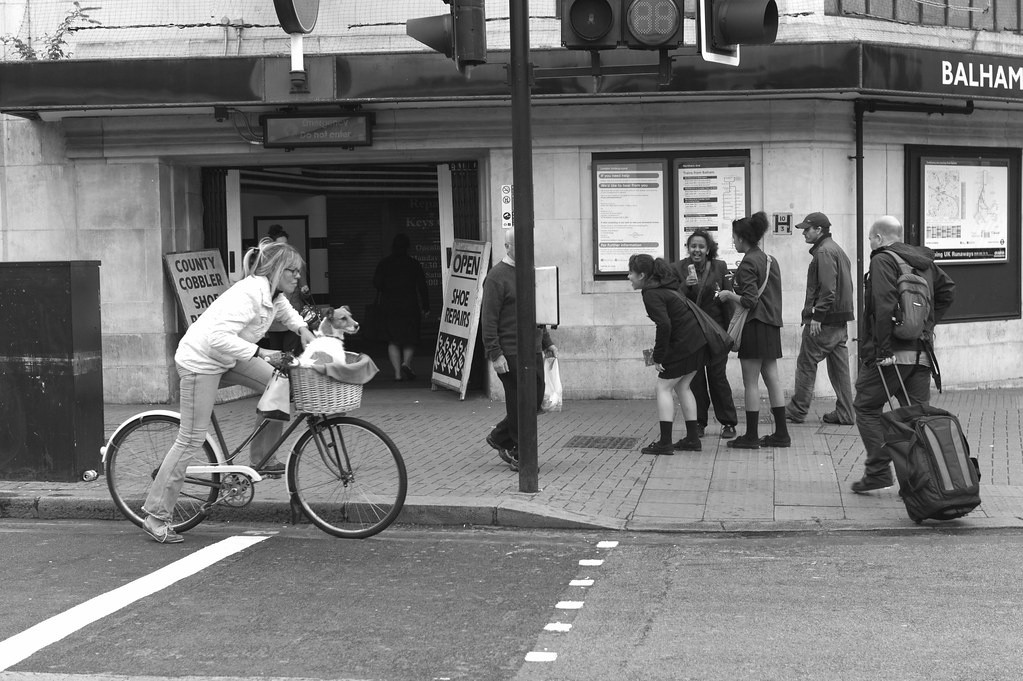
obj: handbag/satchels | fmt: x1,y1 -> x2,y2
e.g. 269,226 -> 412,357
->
256,353 -> 291,422
539,357 -> 562,412
684,297 -> 734,357
726,304 -> 751,352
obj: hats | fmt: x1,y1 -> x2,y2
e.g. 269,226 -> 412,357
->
795,212 -> 831,229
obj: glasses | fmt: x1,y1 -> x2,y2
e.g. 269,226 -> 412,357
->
283,268 -> 302,277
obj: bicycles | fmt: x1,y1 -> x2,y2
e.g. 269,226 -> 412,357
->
100,349 -> 408,540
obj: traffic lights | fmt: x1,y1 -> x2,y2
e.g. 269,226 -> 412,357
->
406,0 -> 488,76
696,0 -> 779,67
560,0 -> 622,50
622,0 -> 685,51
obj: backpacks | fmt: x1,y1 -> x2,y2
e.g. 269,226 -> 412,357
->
879,246 -> 931,343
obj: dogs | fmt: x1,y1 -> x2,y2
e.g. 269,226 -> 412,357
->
296,305 -> 360,365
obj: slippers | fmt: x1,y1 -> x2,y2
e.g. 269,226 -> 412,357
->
401,363 -> 416,379
395,377 -> 403,382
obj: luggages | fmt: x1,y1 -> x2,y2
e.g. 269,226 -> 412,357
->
877,358 -> 982,524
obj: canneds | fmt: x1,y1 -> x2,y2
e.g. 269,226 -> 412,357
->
83,469 -> 98,482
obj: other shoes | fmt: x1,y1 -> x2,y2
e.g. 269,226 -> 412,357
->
850,475 -> 893,492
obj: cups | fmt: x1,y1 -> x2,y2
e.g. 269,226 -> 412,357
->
643,349 -> 654,366
725,274 -> 733,290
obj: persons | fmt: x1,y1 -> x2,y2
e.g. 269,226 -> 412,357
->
627,254 -> 705,454
482,227 -> 558,473
373,233 -> 430,383
267,225 -> 310,402
769,212 -> 856,425
672,230 -> 738,438
141,236 -> 316,544
851,216 -> 956,493
719,211 -> 792,448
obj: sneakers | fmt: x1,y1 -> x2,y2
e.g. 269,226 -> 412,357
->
486,434 -> 512,464
641,441 -> 674,455
721,425 -> 736,438
727,436 -> 761,449
760,435 -> 791,447
141,516 -> 184,543
249,461 -> 286,474
674,437 -> 701,451
697,423 -> 704,437
823,411 -> 840,424
498,447 -> 540,473
771,407 -> 802,424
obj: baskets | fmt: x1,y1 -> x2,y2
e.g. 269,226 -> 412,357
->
287,351 -> 363,415
